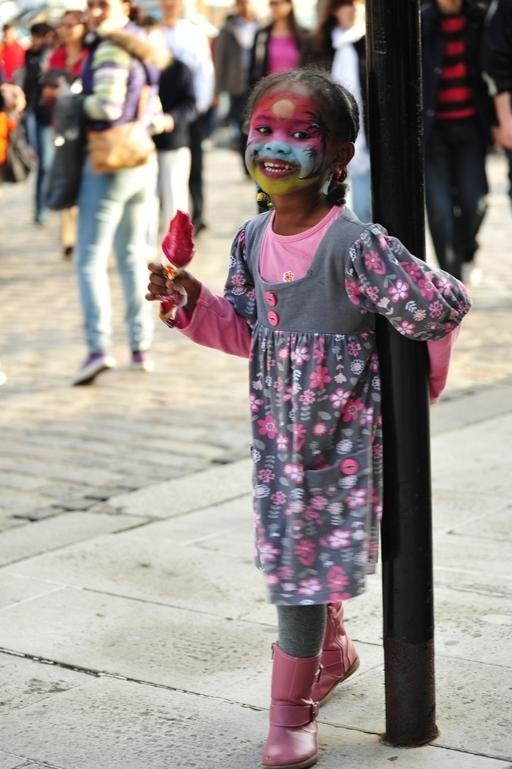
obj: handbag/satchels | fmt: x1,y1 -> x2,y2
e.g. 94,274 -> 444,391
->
83,85 -> 155,173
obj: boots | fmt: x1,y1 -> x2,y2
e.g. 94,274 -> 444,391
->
311,601 -> 360,704
261,642 -> 319,768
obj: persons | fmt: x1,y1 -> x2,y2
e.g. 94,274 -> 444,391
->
2,2 -> 512,384
145,69 -> 472,766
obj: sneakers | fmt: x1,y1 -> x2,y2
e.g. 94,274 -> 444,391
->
71,353 -> 113,386
130,351 -> 150,369
